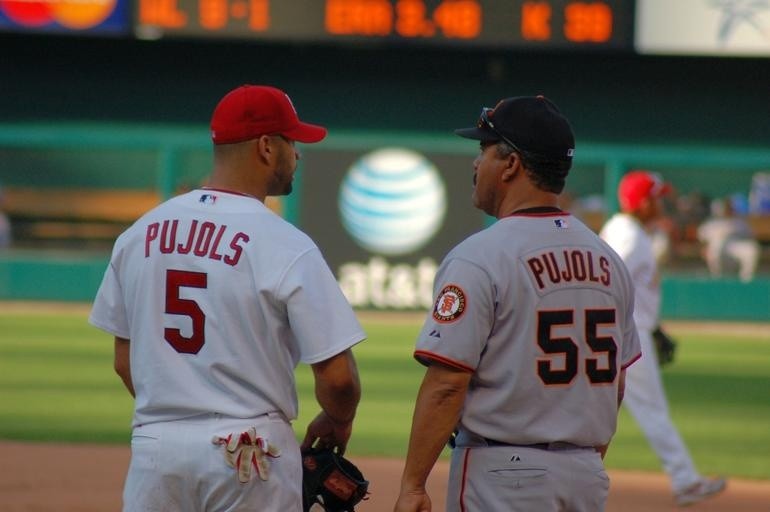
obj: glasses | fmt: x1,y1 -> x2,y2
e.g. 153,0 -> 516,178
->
477,107 -> 525,156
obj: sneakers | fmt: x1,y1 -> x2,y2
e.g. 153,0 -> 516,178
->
673,475 -> 725,506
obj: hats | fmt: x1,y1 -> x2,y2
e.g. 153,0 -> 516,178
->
210,85 -> 328,145
618,170 -> 672,211
453,93 -> 574,170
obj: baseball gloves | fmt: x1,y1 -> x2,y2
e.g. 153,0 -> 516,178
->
653,327 -> 675,362
301,446 -> 371,511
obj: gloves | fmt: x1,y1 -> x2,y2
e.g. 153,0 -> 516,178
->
300,447 -> 370,512
652,328 -> 675,366
211,426 -> 282,484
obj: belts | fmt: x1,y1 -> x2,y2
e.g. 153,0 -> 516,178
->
478,433 -> 553,450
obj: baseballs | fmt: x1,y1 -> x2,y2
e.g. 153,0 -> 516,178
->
336,147 -> 447,256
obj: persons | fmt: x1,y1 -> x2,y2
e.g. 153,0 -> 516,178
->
596,168 -> 732,507
657,170 -> 769,287
81,82 -> 371,510
388,94 -> 646,512
